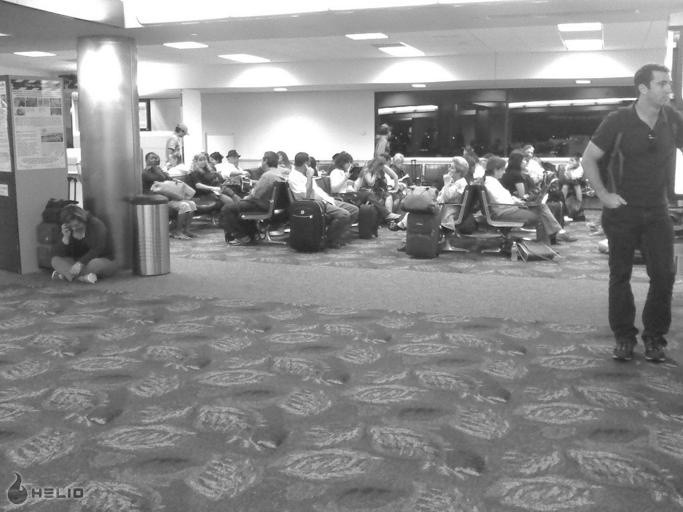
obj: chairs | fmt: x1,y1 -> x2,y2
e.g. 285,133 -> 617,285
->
65,157 -> 588,260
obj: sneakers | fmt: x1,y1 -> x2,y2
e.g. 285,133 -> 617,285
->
79,273 -> 98,284
227,234 -> 253,247
49,270 -> 64,282
610,336 -> 635,362
388,223 -> 407,231
555,230 -> 579,243
172,230 -> 201,240
642,339 -> 667,363
395,244 -> 407,252
563,216 -> 574,222
384,213 -> 401,220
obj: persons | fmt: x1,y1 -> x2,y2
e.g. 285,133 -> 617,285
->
49,205 -> 113,284
583,64 -> 683,363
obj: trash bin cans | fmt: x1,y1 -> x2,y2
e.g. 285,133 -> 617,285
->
132,194 -> 170,277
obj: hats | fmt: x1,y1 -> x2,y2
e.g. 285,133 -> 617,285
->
175,123 -> 192,138
225,149 -> 241,159
